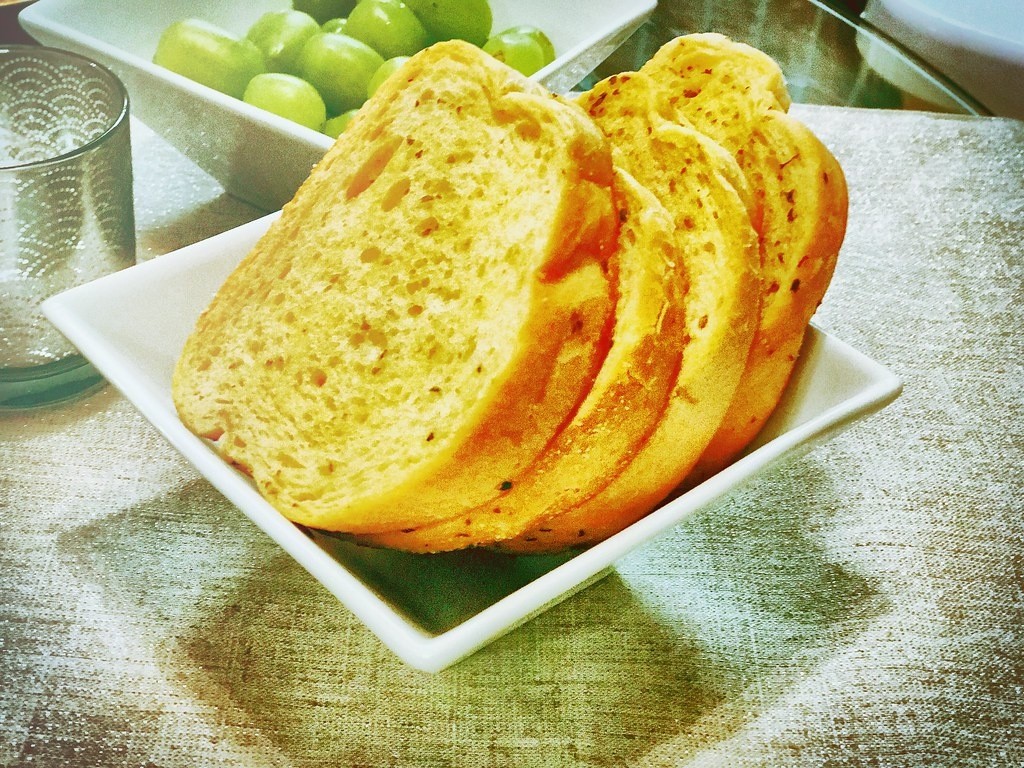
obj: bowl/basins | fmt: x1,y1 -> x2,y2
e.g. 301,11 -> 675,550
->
20,0 -> 661,205
38,208 -> 902,674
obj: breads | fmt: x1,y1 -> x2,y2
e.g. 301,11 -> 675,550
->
336,31 -> 848,554
172,40 -> 621,534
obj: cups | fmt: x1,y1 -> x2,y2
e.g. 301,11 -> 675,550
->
0,43 -> 135,413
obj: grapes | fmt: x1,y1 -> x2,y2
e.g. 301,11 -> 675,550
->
151,0 -> 556,146
